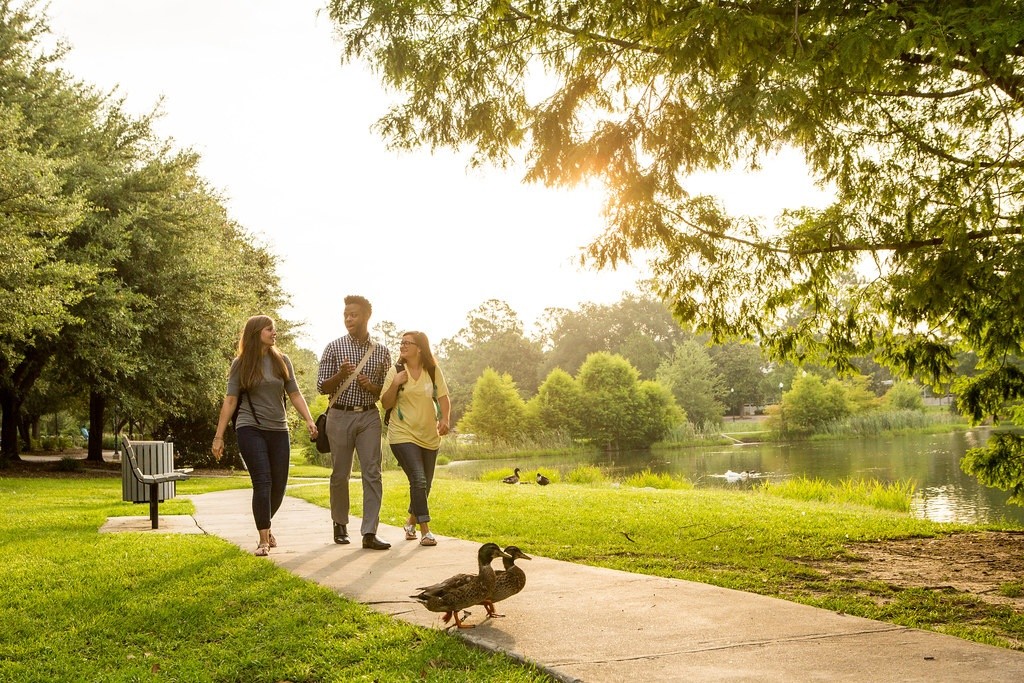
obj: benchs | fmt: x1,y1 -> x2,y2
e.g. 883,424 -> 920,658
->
121,434 -> 192,529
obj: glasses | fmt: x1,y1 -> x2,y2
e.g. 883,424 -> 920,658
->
401,341 -> 418,348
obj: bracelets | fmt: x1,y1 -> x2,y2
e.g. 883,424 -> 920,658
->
214,437 -> 223,440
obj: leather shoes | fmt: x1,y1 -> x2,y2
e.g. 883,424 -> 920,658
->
333,520 -> 350,544
362,533 -> 391,550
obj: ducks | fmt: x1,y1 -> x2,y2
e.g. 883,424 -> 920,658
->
408,543 -> 512,628
503,468 -> 522,484
476,546 -> 532,618
536,473 -> 549,485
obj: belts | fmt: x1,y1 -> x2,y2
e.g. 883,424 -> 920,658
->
332,403 -> 375,412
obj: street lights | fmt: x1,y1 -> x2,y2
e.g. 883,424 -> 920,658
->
780,382 -> 783,403
731,388 -> 735,424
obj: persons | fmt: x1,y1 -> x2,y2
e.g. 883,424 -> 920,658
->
380,332 -> 451,546
212,315 -> 317,554
81,425 -> 88,439
317,296 -> 392,549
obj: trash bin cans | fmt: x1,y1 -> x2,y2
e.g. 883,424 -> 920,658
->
117,441 -> 174,504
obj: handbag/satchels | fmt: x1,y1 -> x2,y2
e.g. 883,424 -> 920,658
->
310,413 -> 331,453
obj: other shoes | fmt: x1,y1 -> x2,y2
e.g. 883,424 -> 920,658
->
254,541 -> 270,555
268,529 -> 277,546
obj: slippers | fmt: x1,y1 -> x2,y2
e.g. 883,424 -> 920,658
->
420,530 -> 437,546
404,524 -> 417,540
